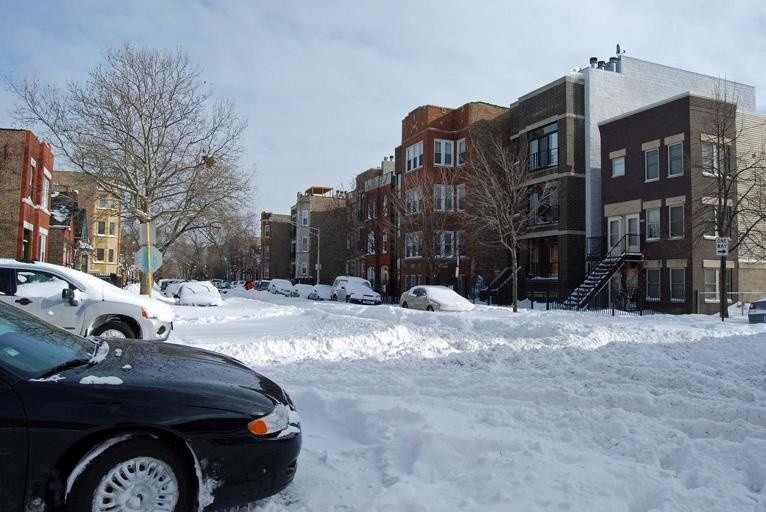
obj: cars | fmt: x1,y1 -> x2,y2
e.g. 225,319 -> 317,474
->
0,297 -> 302,512
223,275 -> 381,305
399,283 -> 472,314
748,299 -> 766,324
159,274 -> 224,307
0,256 -> 174,343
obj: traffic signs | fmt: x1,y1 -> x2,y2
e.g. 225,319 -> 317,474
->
716,236 -> 730,257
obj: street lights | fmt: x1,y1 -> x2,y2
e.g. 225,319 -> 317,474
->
263,216 -> 321,285
